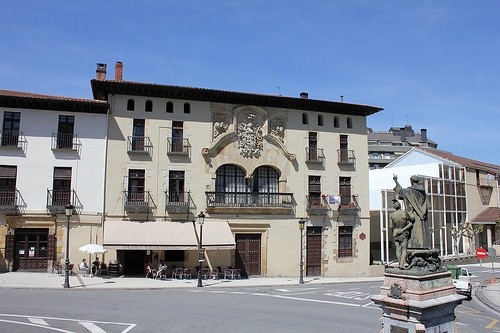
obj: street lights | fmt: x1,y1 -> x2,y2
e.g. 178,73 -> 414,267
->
298,217 -> 306,284
62,202 -> 74,288
197,211 -> 204,287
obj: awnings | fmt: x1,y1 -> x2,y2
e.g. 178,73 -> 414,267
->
102,218 -> 236,252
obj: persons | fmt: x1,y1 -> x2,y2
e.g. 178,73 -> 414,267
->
79,259 -> 91,276
90,258 -> 101,276
393,173 -> 433,249
144,259 -> 168,279
388,200 -> 413,270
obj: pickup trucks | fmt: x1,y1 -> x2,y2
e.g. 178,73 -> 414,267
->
447,267 -> 473,301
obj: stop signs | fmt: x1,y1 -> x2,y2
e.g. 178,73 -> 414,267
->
476,248 -> 487,259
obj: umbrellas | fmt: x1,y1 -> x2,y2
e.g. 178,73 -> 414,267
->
78,244 -> 107,276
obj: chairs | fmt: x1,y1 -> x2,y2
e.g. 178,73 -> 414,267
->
78,263 -> 108,275
201,267 -> 241,279
145,266 -> 192,280
